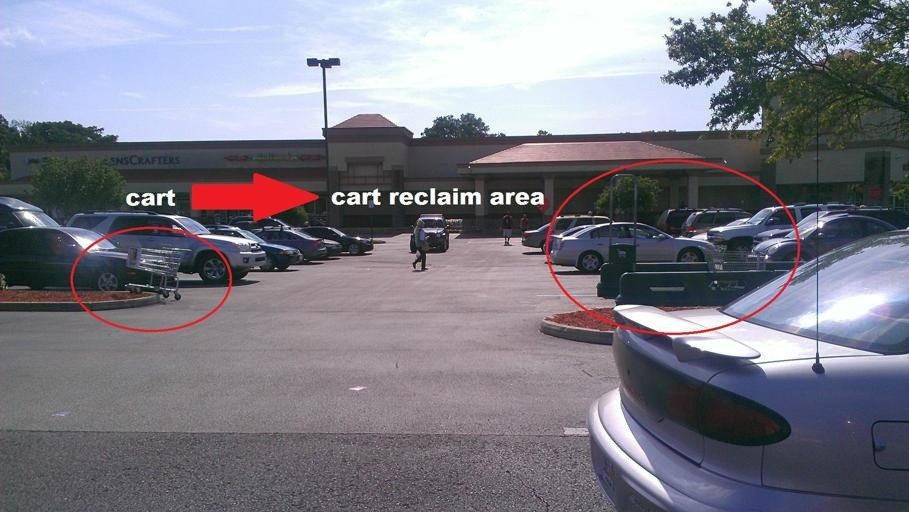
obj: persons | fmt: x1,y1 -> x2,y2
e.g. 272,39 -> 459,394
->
501,210 -> 513,246
586,208 -> 595,217
519,214 -> 530,242
412,217 -> 431,271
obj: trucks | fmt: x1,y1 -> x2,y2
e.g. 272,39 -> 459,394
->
0,195 -> 59,229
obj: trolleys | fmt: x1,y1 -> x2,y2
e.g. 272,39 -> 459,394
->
123,242 -> 194,301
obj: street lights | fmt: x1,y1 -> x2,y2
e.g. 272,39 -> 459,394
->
307,57 -> 343,230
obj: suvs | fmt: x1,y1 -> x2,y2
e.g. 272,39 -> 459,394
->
657,204 -> 909,262
408,212 -> 453,255
67,207 -> 269,289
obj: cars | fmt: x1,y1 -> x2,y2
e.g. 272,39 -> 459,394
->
584,227 -> 909,512
750,211 -> 909,272
518,212 -> 618,255
550,221 -> 717,274
1,225 -> 136,293
202,222 -> 304,273
541,225 -> 592,253
230,214 -> 377,259
